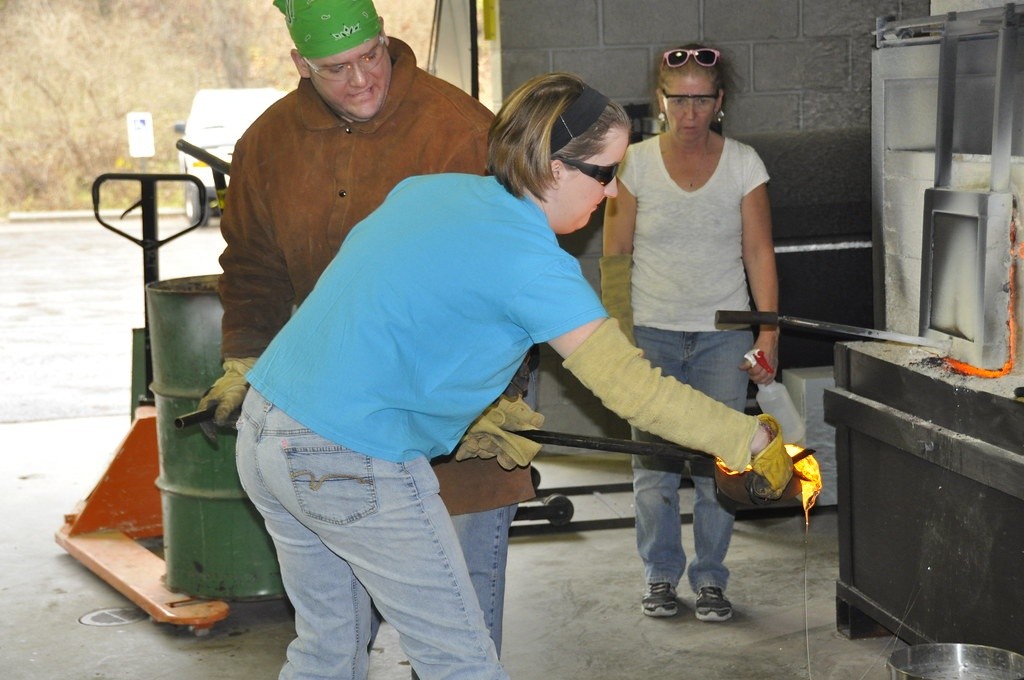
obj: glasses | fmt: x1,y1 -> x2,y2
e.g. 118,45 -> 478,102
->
551,155 -> 620,185
296,31 -> 383,80
659,47 -> 722,74
660,87 -> 720,110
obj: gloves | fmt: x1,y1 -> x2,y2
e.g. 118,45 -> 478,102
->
453,393 -> 545,471
197,356 -> 259,445
750,412 -> 792,500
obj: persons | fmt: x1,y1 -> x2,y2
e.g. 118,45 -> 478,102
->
597,43 -> 782,623
195,0 -> 536,680
236,75 -> 793,680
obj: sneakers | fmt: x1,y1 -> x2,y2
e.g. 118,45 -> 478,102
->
695,585 -> 733,622
641,580 -> 678,617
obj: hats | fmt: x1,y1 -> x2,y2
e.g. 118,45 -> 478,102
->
272,0 -> 381,57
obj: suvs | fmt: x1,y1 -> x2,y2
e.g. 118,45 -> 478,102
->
173,86 -> 291,227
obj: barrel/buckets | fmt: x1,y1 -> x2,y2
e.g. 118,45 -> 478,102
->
145,275 -> 285,600
888,642 -> 1023,679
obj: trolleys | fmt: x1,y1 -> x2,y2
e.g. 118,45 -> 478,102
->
52,172 -> 232,637
